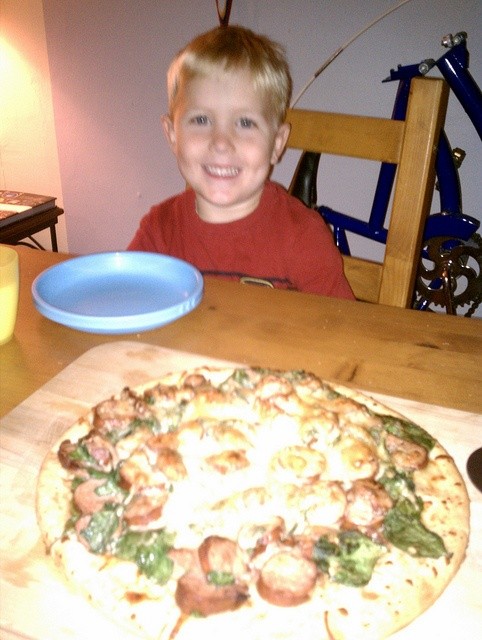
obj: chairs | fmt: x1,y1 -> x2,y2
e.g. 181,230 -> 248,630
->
268,77 -> 451,309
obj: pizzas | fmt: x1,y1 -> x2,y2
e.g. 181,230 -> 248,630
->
35,365 -> 473,640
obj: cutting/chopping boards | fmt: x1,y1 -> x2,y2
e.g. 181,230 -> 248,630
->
0,339 -> 482,640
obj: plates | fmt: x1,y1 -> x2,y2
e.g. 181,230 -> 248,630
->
30,249 -> 206,336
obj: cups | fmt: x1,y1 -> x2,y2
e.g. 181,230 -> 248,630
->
0,243 -> 20,349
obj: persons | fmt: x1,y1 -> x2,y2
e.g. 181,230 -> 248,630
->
126,24 -> 355,299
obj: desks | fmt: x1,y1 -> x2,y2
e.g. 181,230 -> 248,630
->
0,191 -> 64,255
